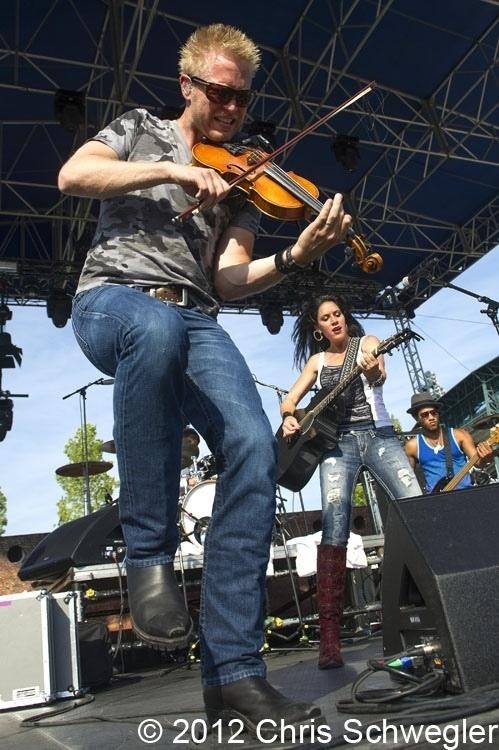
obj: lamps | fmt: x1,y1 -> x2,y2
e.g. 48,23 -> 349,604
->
55,89 -> 86,135
46,288 -> 74,327
250,122 -> 283,164
331,132 -> 360,174
260,298 -> 288,335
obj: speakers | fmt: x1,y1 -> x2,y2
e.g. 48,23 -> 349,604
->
17,507 -> 126,581
381,485 -> 498,696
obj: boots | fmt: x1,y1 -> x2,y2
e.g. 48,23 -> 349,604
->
317,544 -> 348,670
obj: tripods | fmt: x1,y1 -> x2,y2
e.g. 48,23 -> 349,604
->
161,494 -> 320,681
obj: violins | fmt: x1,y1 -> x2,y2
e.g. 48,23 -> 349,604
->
192,134 -> 384,275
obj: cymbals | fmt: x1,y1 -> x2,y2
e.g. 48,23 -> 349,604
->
97,440 -> 116,454
180,436 -> 199,470
56,460 -> 113,476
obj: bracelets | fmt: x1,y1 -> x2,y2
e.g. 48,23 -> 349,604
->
282,411 -> 292,423
275,247 -> 295,275
367,370 -> 385,388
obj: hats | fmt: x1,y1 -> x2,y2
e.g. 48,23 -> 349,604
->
407,394 -> 444,414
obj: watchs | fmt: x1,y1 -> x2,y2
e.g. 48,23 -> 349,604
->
286,245 -> 310,272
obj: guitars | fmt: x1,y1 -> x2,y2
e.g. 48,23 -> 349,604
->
431,423 -> 499,494
275,329 -> 414,491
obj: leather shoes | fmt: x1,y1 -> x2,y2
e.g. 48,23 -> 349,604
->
202,675 -> 325,739
126,561 -> 194,646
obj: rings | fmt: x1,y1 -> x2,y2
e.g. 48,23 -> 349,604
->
363,363 -> 368,367
326,221 -> 334,226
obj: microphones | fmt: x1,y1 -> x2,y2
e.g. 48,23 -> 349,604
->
382,258 -> 438,307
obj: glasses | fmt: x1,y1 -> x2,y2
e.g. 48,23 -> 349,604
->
191,76 -> 256,108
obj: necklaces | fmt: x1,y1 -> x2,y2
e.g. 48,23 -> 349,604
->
423,429 -> 444,451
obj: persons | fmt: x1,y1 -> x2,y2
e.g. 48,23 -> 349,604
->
55,18 -> 354,741
276,287 -> 427,672
402,392 -> 494,498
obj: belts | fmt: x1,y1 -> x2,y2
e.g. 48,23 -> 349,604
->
131,284 -> 201,307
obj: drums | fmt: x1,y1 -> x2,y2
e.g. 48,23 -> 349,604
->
181,477 -> 217,547
196,454 -> 218,481
